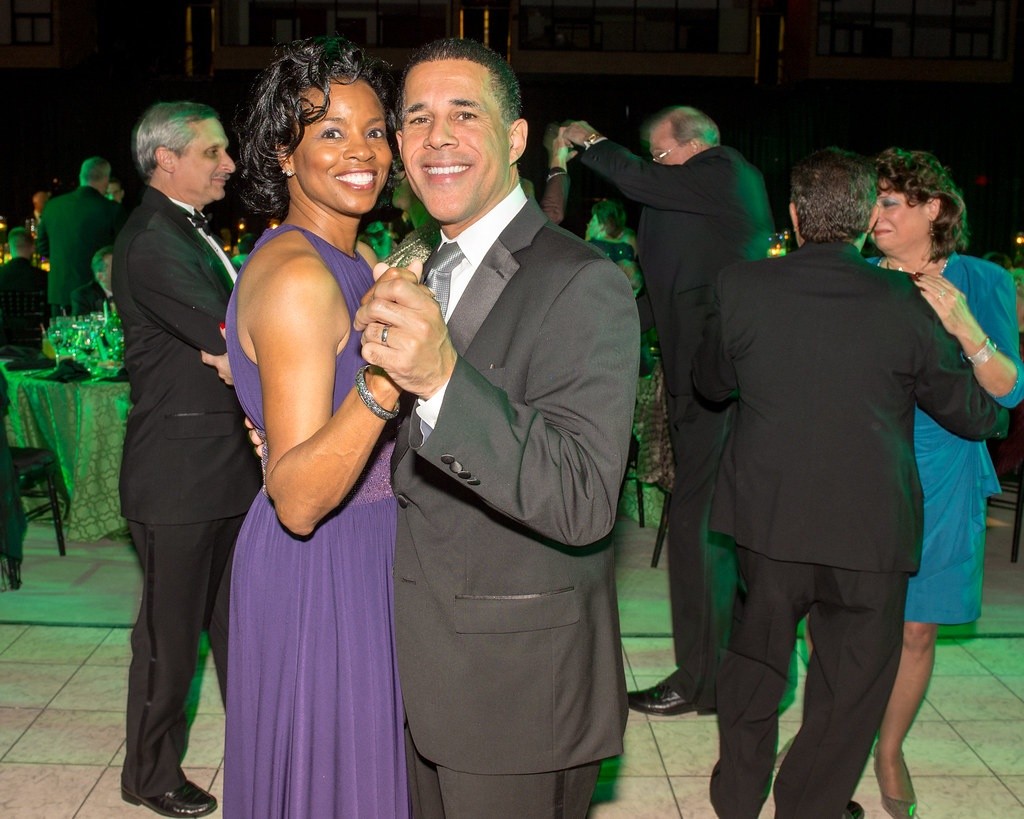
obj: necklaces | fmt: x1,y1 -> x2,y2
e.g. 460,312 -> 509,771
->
875,256 -> 949,276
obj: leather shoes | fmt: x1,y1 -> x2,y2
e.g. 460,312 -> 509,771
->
120,778 -> 217,819
626,678 -> 720,716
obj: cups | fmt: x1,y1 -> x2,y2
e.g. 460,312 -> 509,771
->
767,234 -> 786,258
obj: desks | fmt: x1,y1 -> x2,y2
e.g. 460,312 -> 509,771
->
0,350 -> 134,544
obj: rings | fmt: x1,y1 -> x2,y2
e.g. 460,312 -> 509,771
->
381,324 -> 391,347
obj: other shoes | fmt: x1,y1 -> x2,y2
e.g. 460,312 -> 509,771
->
843,798 -> 867,819
871,743 -> 917,817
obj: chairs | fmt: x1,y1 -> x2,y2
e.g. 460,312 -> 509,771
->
986,457 -> 1024,562
0,374 -> 66,590
617,437 -> 672,568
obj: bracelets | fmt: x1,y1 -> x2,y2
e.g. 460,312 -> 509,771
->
354,364 -> 401,421
584,133 -> 604,143
546,171 -> 568,182
960,336 -> 997,368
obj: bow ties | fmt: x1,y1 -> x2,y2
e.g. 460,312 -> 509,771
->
191,207 -> 214,236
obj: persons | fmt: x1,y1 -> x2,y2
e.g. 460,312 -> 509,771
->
0,102 -> 1024,717
691,145 -> 1024,819
225,32 -> 436,819
109,101 -> 265,819
352,35 -> 644,819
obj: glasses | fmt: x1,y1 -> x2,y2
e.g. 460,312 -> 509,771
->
651,145 -> 681,162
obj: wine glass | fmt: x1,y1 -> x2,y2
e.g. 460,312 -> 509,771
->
42,311 -> 122,374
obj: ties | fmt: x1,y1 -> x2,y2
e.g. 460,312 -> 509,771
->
424,241 -> 466,322
